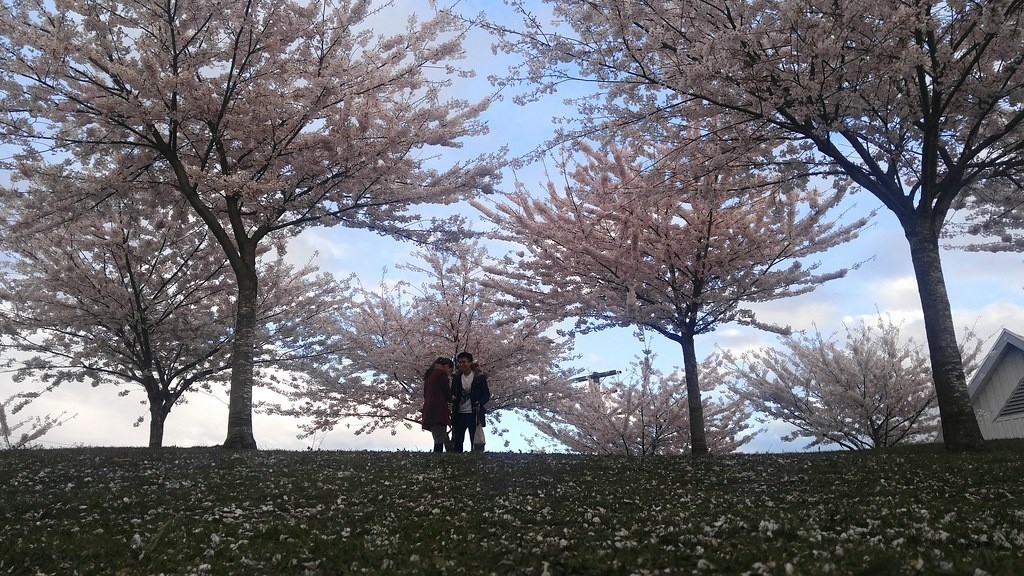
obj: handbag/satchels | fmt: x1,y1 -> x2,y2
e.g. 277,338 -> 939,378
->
473,423 -> 486,445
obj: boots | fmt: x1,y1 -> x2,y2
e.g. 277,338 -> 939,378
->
434,443 -> 443,452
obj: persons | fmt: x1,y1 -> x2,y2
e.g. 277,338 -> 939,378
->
451,352 -> 489,452
422,357 -> 457,452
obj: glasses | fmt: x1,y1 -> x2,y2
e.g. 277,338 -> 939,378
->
458,361 -> 469,364
